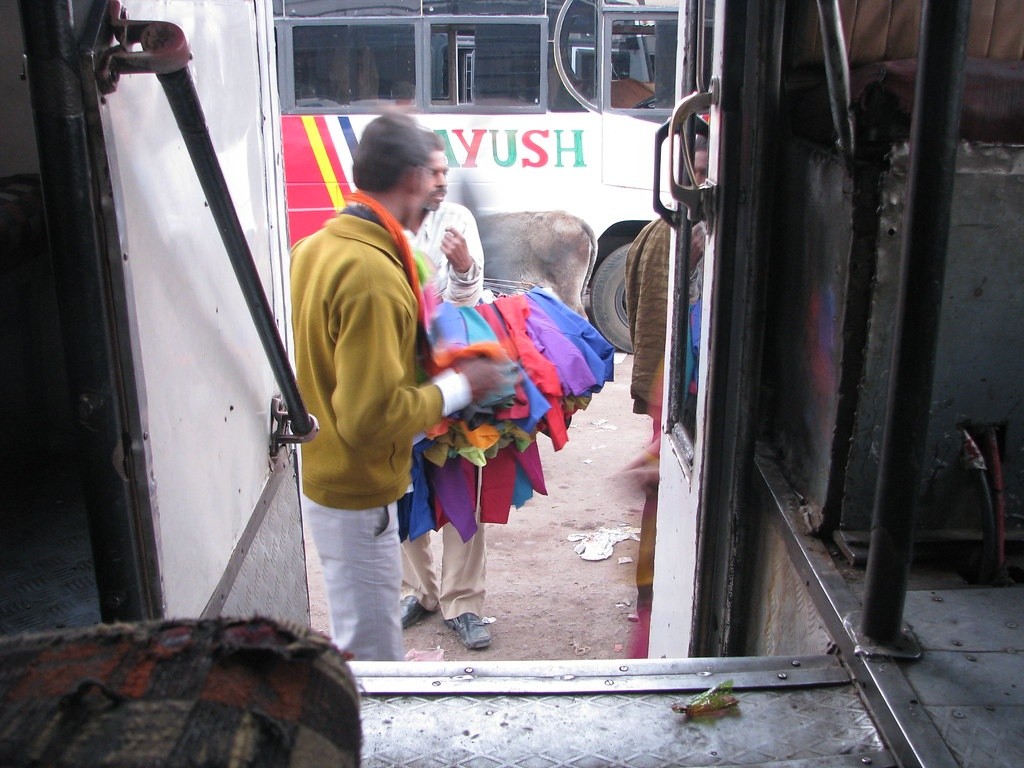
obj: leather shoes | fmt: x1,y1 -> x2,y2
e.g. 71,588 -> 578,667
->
400,595 -> 439,628
444,613 -> 491,648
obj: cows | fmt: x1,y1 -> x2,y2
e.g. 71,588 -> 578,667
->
471,211 -> 598,324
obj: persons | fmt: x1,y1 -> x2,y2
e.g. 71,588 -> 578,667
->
289,114 -> 506,662
388,130 -> 715,654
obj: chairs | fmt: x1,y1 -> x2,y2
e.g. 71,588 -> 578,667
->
333,42 -> 380,104
0,611 -> 361,768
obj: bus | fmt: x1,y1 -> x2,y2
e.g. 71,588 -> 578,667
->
265,2 -> 700,357
